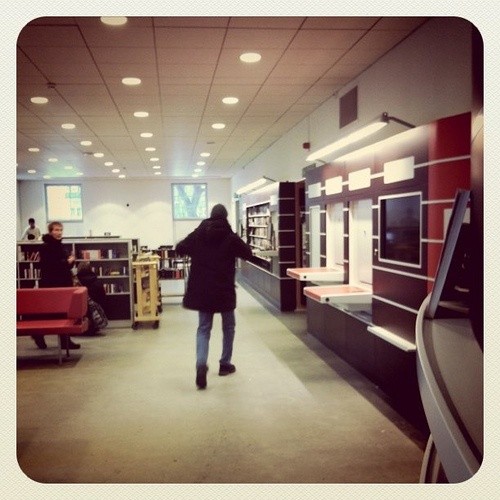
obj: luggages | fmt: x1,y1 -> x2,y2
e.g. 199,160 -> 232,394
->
75,283 -> 108,334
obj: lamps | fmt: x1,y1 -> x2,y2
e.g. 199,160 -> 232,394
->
304,112 -> 389,162
235,176 -> 267,194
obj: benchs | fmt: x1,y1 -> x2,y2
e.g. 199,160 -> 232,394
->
16,286 -> 88,365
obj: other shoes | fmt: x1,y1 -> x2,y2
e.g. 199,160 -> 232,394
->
31,335 -> 48,348
219,363 -> 235,376
196,367 -> 208,389
60,337 -> 81,349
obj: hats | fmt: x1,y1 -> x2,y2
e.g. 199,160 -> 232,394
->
211,204 -> 227,215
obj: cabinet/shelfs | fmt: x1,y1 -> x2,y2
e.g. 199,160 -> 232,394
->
132,239 -> 138,304
240,181 -> 297,312
16,239 -> 133,328
306,289 -> 429,431
142,249 -> 191,280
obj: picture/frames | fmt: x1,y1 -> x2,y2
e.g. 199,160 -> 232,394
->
171,183 -> 208,221
44,183 -> 84,223
